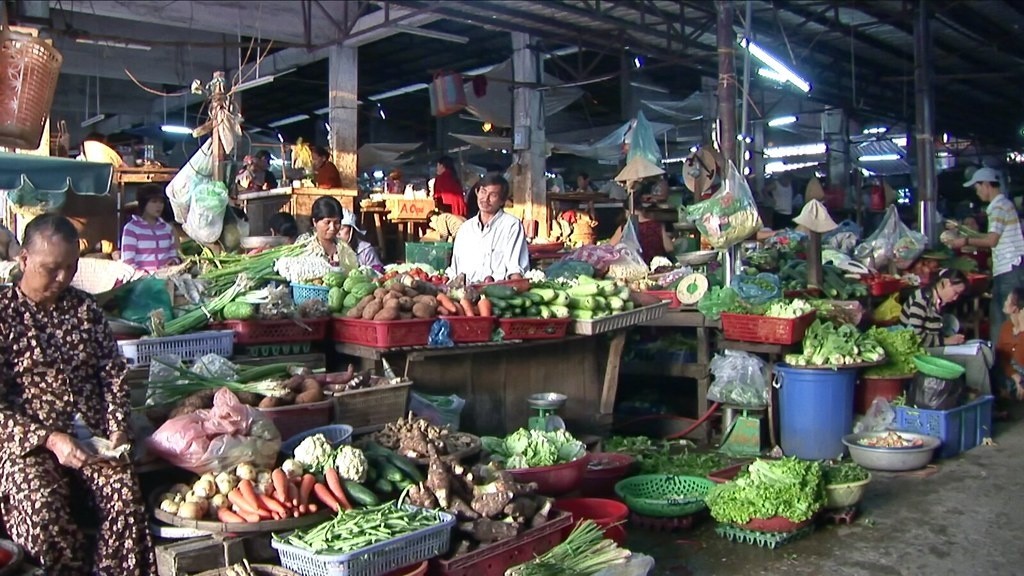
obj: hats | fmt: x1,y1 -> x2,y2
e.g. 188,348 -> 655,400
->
341,207 -> 361,230
681,150 -> 716,194
963,167 -> 1001,188
135,182 -> 166,201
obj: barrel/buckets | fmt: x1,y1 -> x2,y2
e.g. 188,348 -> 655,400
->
771,364 -> 857,461
551,498 -> 628,549
584,452 -> 634,497
855,375 -> 914,417
381,559 -> 429,576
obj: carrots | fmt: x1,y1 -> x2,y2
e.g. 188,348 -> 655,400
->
434,293 -> 492,318
215,466 -> 352,524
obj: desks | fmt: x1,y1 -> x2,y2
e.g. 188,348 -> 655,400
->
360,207 -> 385,258
392,219 -> 427,256
715,343 -> 791,447
632,313 -> 724,442
118,167 -> 180,224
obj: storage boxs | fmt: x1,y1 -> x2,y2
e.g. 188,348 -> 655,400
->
395,199 -> 435,219
115,232 -> 992,576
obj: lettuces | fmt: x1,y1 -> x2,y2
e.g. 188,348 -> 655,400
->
479,427 -> 586,469
703,455 -> 828,523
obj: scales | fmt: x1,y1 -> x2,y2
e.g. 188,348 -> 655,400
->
710,403 -> 769,457
676,249 -> 718,310
525,391 -> 570,433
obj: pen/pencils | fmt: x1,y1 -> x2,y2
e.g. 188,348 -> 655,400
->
952,328 -> 958,335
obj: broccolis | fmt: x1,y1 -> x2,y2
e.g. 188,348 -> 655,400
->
292,432 -> 369,483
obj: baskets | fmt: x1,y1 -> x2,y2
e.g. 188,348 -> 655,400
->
272,504 -> 457,576
0,22 -> 64,150
614,474 -> 717,516
211,316 -> 329,345
288,283 -> 330,308
332,316 -> 568,347
282,424 -> 355,456
116,327 -> 234,367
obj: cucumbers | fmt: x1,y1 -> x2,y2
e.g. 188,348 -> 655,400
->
480,282 -> 547,319
340,440 -> 423,507
777,259 -> 869,300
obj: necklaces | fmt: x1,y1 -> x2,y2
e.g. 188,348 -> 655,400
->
1011,345 -> 1016,352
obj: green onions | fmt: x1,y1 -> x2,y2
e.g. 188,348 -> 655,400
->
202,233 -> 316,291
136,356 -> 293,399
162,272 -> 249,335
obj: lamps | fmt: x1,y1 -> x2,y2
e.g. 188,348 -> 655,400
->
80,76 -> 104,127
737,31 -> 812,93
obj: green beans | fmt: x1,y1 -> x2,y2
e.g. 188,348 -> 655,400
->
271,499 -> 443,554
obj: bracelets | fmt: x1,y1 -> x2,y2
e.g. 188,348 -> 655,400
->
965,236 -> 969,246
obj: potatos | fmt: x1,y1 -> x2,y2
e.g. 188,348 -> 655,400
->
348,282 -> 439,322
155,461 -> 273,519
143,363 -> 355,414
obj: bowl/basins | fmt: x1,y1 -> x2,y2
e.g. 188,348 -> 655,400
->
471,277 -> 532,294
914,356 -> 965,380
502,450 -> 591,494
68,257 -> 134,306
842,431 -> 942,471
729,513 -> 816,532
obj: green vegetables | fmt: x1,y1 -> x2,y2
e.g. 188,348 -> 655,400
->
530,277 -> 573,291
735,279 -> 928,375
504,516 -> 631,576
822,461 -> 869,484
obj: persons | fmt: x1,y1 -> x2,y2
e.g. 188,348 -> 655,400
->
610,193 -> 675,271
990,286 -> 1024,401
121,184 -> 180,274
202,205 -> 249,257
300,195 -> 359,285
0,211 -> 157,576
952,214 -> 991,272
386,155 -> 506,243
248,212 -> 297,256
451,171 -> 530,285
642,176 -> 668,202
235,150 -> 279,193
75,133 -> 108,161
948,166 -> 1024,345
897,268 -> 1008,421
805,169 -> 827,204
764,161 -> 793,232
312,147 -> 343,187
336,207 -> 385,276
575,172 -> 593,193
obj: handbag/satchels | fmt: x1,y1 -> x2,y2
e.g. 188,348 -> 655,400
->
626,109 -> 662,167
164,137 -> 228,243
861,202 -> 928,268
683,157 -> 762,251
